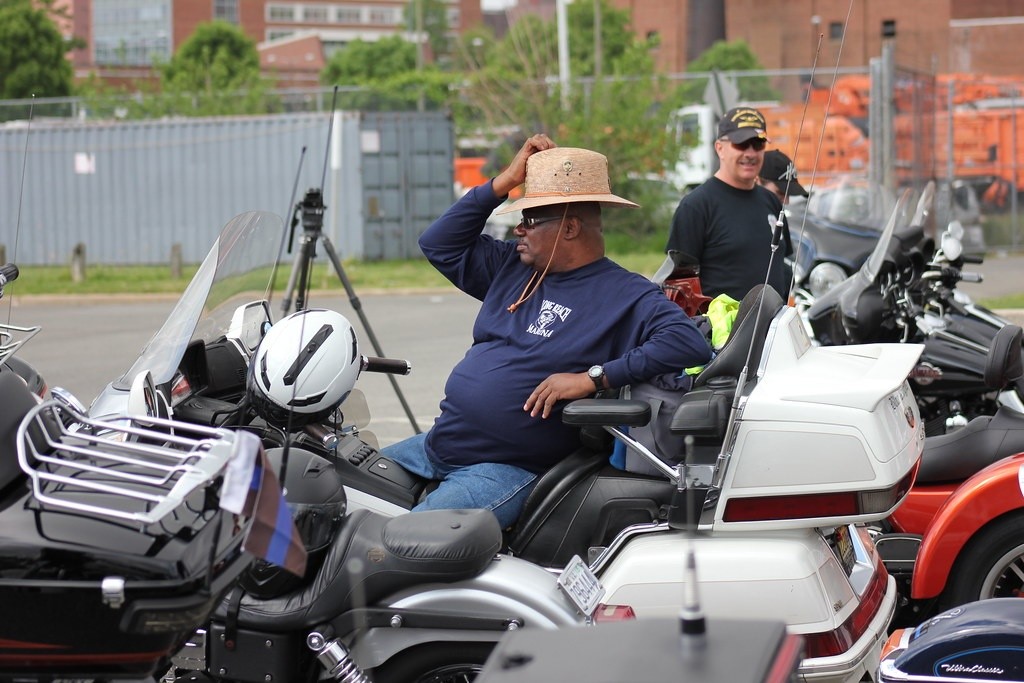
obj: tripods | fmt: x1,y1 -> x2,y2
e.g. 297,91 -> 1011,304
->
280,189 -> 421,435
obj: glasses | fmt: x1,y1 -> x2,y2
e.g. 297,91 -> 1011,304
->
719,137 -> 767,152
521,211 -> 585,229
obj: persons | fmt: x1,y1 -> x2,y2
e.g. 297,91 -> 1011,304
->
664,106 -> 795,307
376,132 -> 716,532
755,149 -> 809,206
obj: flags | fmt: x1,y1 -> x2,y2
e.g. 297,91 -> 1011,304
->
218,430 -> 307,576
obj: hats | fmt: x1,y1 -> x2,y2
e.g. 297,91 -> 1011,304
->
759,149 -> 809,198
718,106 -> 772,145
495,147 -> 641,215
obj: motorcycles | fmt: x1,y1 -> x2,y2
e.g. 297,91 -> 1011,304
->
1,177 -> 1024,682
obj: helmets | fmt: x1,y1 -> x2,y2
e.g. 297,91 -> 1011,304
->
246,308 -> 363,434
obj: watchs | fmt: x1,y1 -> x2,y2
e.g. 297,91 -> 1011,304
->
588,365 -> 608,393
